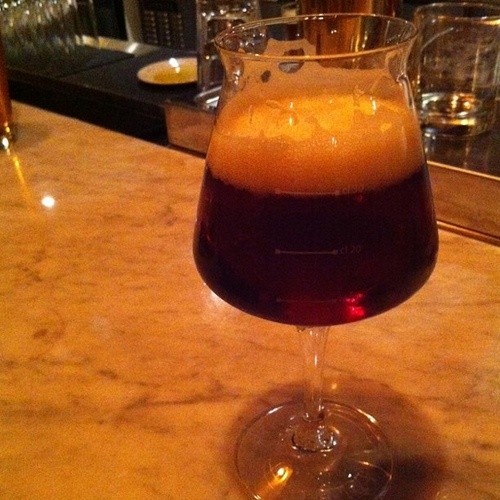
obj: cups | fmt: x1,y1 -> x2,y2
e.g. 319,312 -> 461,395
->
280,2 -> 299,56
0,31 -> 16,148
194,0 -> 266,111
412,2 -> 500,139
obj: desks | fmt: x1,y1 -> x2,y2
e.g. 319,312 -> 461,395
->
0,102 -> 500,500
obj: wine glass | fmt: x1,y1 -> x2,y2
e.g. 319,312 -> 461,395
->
192,12 -> 439,500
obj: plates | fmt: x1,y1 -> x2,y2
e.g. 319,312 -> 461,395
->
137,57 -> 198,86
